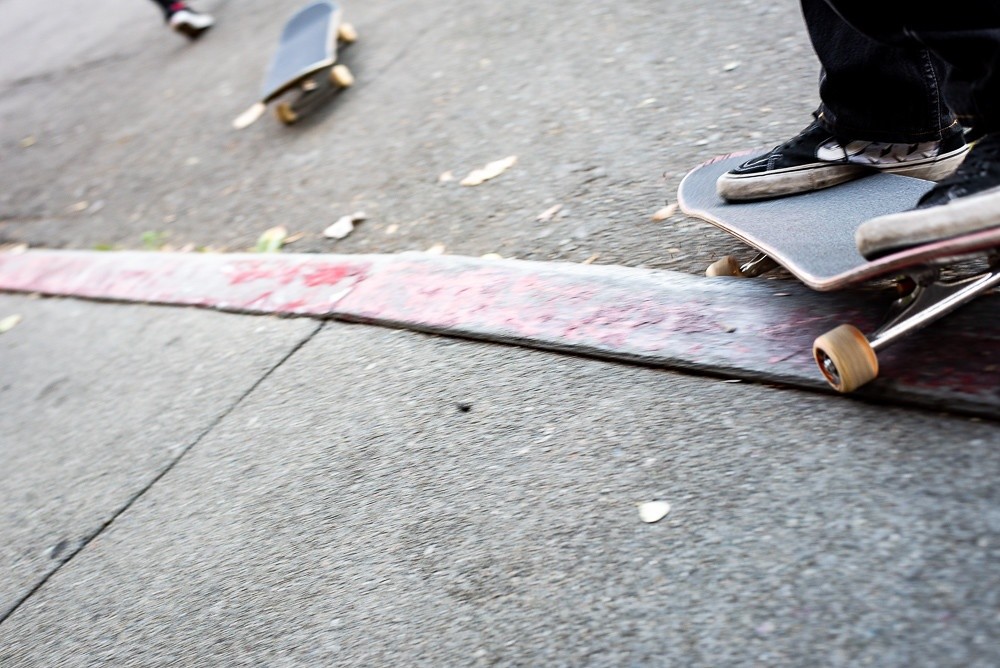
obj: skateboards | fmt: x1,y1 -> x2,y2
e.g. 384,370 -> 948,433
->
257,0 -> 359,128
675,146 -> 1000,394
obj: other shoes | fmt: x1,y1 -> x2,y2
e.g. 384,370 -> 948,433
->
166,8 -> 214,32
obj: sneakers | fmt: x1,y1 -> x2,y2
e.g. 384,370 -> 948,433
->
717,120 -> 970,198
855,136 -> 1000,257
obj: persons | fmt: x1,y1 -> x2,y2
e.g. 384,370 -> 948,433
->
715,0 -> 1000,262
154,0 -> 215,36
